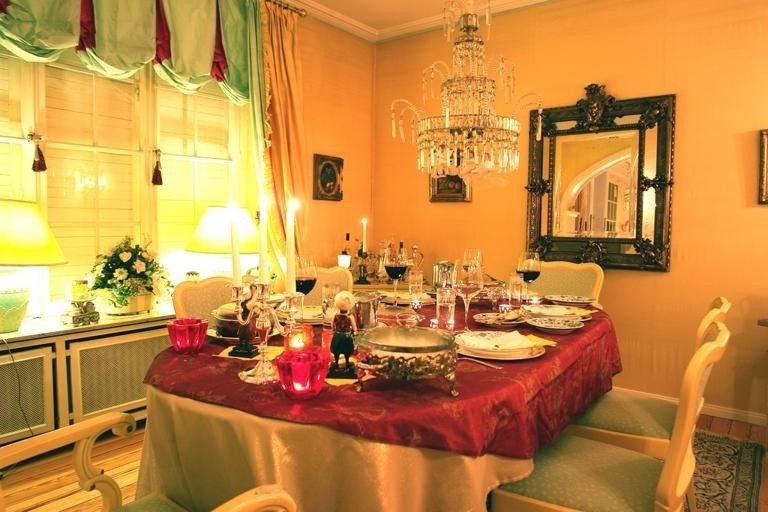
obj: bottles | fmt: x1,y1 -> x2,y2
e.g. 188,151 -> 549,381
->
342,232 -> 375,281
397,241 -> 405,252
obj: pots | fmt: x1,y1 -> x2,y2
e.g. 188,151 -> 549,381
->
353,319 -> 461,397
211,301 -> 274,335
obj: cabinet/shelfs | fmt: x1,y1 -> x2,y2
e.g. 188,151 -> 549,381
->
0,314 -> 177,446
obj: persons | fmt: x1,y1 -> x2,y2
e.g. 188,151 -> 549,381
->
329,290 -> 356,369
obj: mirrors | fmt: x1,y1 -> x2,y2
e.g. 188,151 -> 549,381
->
524,81 -> 679,273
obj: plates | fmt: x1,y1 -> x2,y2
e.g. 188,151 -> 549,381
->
524,294 -> 597,335
201,326 -> 281,344
291,304 -> 333,325
472,311 -> 522,328
452,330 -> 545,360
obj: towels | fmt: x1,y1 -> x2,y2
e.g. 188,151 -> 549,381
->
458,326 -> 561,361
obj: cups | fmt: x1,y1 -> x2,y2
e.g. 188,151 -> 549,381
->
166,317 -> 209,354
405,269 -> 423,309
321,281 -> 339,328
275,348 -> 332,400
507,272 -> 523,306
435,287 -> 456,329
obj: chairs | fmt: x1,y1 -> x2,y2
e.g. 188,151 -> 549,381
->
294,271 -> 358,301
487,320 -> 731,510
568,295 -> 733,510
168,278 -> 239,325
0,407 -> 297,511
507,260 -> 607,307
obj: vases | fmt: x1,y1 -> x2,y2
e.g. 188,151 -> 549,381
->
96,290 -> 156,315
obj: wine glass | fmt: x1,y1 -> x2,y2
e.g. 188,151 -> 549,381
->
482,281 -> 503,311
383,252 -> 407,305
451,259 -> 483,330
516,250 -> 542,302
294,254 -> 317,329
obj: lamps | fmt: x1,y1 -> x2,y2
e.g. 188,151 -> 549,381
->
391,0 -> 542,174
0,195 -> 70,338
182,206 -> 267,296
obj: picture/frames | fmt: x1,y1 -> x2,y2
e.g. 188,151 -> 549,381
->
312,153 -> 344,202
427,150 -> 471,204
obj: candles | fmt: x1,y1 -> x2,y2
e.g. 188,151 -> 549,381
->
258,208 -> 266,281
361,220 -> 371,253
230,224 -> 244,288
285,196 -> 304,296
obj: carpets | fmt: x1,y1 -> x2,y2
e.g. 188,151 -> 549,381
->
680,428 -> 766,512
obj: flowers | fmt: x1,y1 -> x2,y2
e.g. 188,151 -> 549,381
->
82,232 -> 172,305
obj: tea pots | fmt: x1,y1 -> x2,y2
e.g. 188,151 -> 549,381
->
373,254 -> 388,282
353,293 -> 383,328
407,244 -> 425,270
